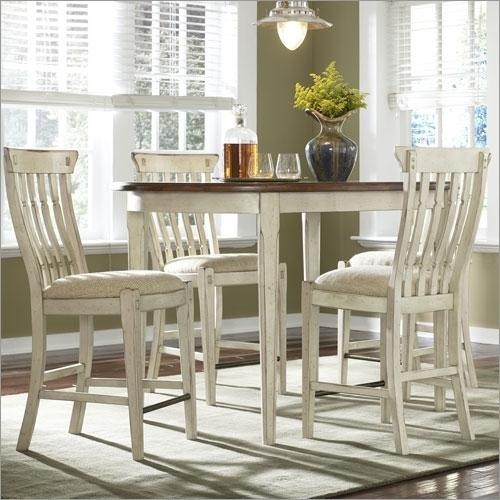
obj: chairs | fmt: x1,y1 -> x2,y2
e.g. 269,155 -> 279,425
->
3,147 -> 491,461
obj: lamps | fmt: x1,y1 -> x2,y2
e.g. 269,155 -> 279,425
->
250,2 -> 333,50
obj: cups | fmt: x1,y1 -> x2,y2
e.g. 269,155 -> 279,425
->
275,153 -> 303,181
247,151 -> 275,179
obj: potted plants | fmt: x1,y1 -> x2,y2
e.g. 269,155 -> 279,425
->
293,59 -> 367,181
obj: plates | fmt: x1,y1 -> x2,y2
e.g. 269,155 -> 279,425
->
212,175 -> 306,183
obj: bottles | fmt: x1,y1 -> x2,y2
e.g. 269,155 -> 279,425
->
222,102 -> 258,180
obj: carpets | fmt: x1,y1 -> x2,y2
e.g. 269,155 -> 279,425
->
1,354 -> 499,498
